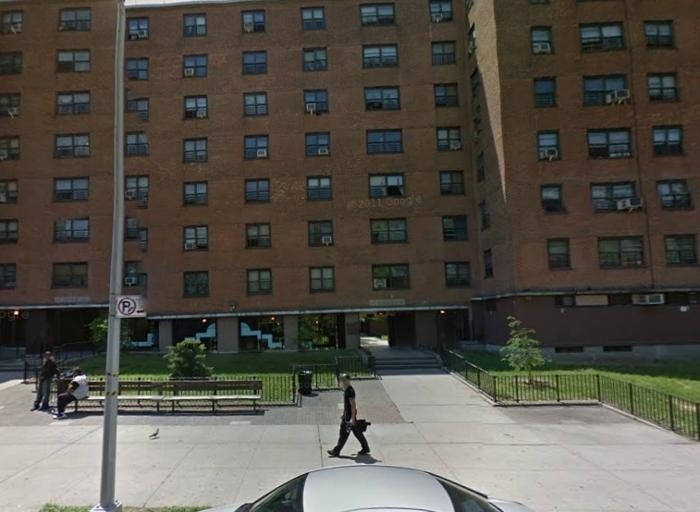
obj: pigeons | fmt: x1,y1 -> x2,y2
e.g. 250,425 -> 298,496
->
149,429 -> 158,437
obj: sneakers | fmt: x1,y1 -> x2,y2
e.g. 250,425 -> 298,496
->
31,406 -> 65,418
327,449 -> 339,456
358,449 -> 369,454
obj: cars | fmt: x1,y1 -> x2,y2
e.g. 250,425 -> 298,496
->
200,465 -> 530,512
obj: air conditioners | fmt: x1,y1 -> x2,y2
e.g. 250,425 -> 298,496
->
607,87 -> 643,214
304,102 -> 332,245
531,39 -> 559,161
256,147 -> 267,158
125,189 -> 135,286
8,22 -> 22,116
181,65 -> 207,251
137,29 -> 148,39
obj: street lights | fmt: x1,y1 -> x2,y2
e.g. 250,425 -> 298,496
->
13,309 -> 19,360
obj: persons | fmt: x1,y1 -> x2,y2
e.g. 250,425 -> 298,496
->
30,351 -> 59,412
327,372 -> 369,458
52,366 -> 88,419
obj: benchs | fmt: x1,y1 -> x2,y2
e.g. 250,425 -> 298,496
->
73,380 -> 263,414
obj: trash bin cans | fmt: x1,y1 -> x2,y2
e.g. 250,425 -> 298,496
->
298,371 -> 313,396
